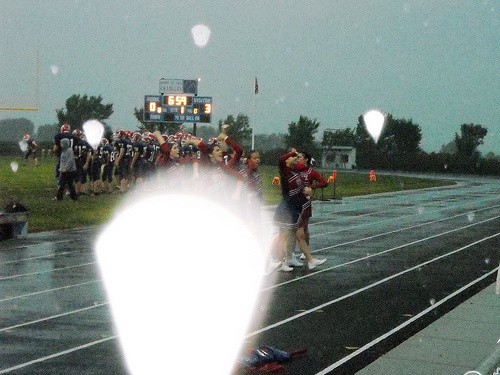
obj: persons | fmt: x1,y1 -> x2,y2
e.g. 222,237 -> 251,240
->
273,145 -> 326,272
286,146 -> 297,152
152,129 -> 186,192
272,152 -> 328,259
53,122 -> 263,204
114,129 -> 132,194
22,131 -> 39,166
131,132 -> 146,193
56,138 -> 80,201
184,133 -> 243,198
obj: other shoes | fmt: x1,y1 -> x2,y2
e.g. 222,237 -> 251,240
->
56,184 -> 130,200
285,258 -> 303,267
299,252 -> 307,259
308,258 -> 327,269
275,261 -> 293,271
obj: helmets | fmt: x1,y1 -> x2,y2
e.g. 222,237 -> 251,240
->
60,124 -> 229,145
